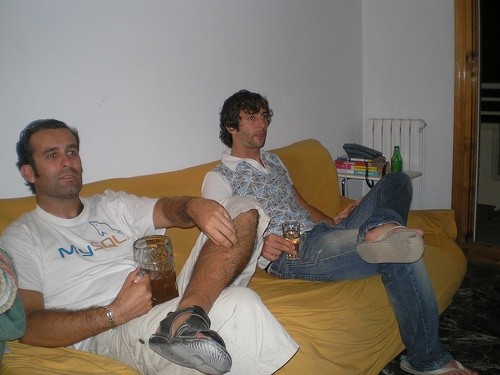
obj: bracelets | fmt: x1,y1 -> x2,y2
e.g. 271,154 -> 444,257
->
103,306 -> 118,330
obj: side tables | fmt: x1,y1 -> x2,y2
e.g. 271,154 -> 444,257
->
337,169 -> 423,197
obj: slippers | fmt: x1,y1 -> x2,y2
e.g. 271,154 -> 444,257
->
356,225 -> 426,265
399,357 -> 464,375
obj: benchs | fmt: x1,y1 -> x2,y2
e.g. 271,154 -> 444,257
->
0,137 -> 470,375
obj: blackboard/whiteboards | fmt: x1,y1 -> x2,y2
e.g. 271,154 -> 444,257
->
344,144 -> 382,159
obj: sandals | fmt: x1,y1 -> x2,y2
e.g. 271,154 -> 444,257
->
148,304 -> 233,375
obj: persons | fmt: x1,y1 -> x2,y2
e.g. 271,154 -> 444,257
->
0,119 -> 301,375
202,89 -> 480,375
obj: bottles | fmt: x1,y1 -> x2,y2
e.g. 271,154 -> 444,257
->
391,146 -> 403,172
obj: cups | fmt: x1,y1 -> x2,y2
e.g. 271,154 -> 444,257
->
133,234 -> 180,305
283,221 -> 301,260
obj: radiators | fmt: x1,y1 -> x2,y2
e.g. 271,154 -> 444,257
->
364,117 -> 427,210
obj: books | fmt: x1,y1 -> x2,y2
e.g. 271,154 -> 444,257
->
334,153 -> 386,177
